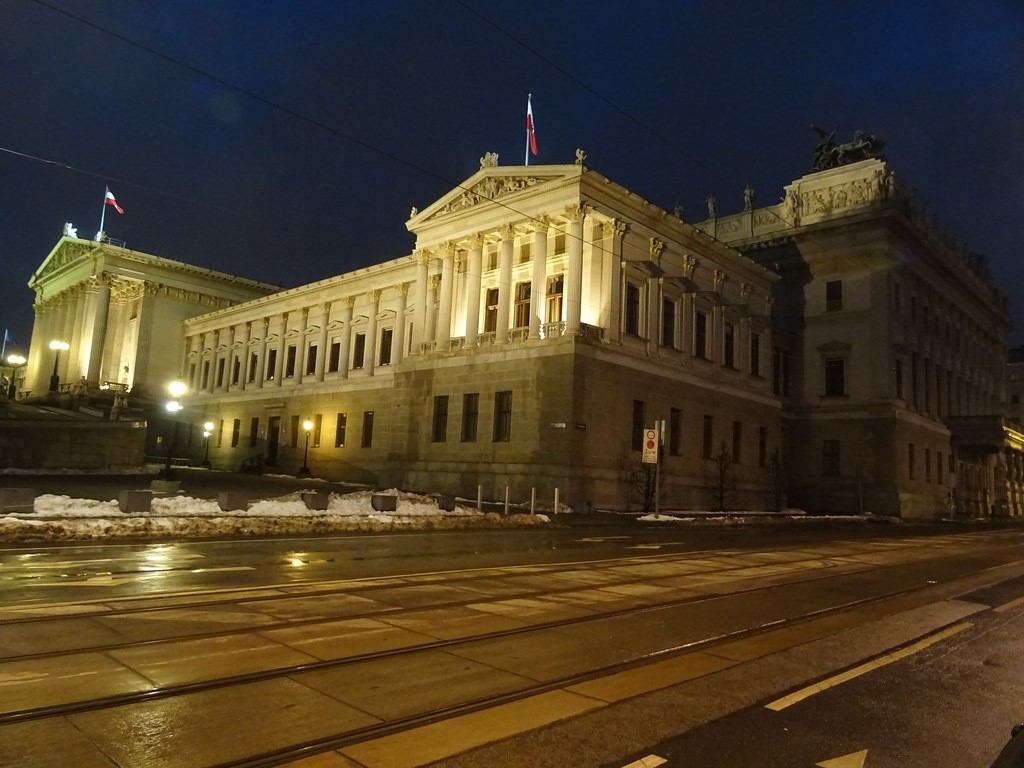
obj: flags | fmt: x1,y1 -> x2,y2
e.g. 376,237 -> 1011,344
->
527,100 -> 538,154
7,332 -> 16,345
105,188 -> 124,214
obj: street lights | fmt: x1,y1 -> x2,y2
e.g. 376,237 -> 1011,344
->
201,421 -> 213,467
6,354 -> 27,399
162,376 -> 188,471
47,338 -> 70,392
300,419 -> 313,473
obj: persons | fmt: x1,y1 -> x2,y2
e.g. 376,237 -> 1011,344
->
0,372 -> 8,387
